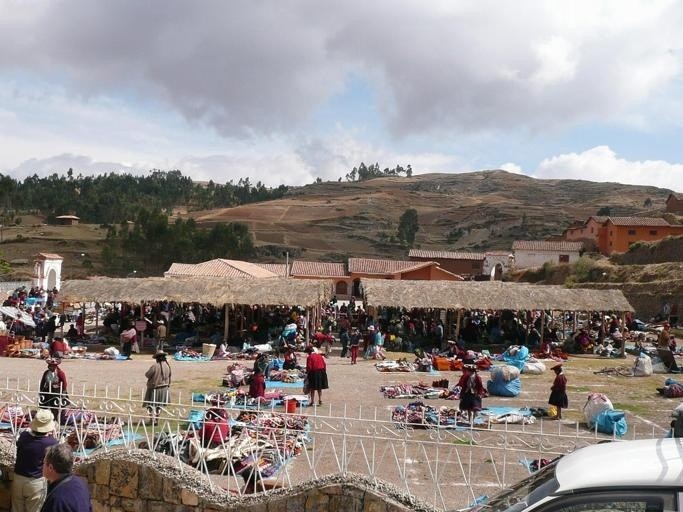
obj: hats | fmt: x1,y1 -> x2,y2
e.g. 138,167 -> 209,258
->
550,364 -> 562,370
151,349 -> 169,359
28,408 -> 55,433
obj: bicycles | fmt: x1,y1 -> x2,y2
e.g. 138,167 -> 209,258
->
592,364 -> 633,377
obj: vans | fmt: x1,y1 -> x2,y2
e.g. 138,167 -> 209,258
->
455,438 -> 683,512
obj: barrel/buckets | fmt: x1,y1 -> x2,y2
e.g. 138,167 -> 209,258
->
201,343 -> 216,358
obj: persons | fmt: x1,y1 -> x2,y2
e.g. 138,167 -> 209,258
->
458,364 -> 486,426
658,322 -> 683,373
315,295 -> 446,367
441,308 -> 648,360
247,352 -> 271,399
196,405 -> 228,447
3,284 -> 88,356
141,350 -> 174,425
39,356 -> 70,423
40,442 -> 93,512
11,409 -> 59,512
304,344 -> 329,406
547,364 -> 568,420
100,303 -> 305,370
